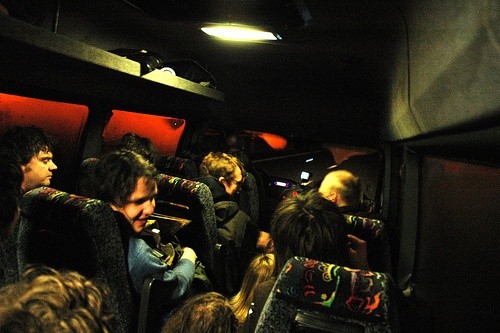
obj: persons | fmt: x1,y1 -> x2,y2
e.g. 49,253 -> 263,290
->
0,124 -> 388,333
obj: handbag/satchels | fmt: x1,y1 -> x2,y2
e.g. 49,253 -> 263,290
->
107,48 -> 163,75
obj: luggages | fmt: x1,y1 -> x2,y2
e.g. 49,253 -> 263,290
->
167,59 -> 216,90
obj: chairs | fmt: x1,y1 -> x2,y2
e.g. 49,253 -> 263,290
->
236,172 -> 261,224
252,255 -> 402,333
17,184 -> 168,333
159,155 -> 197,180
345,213 -> 386,243
156,173 -> 227,296
